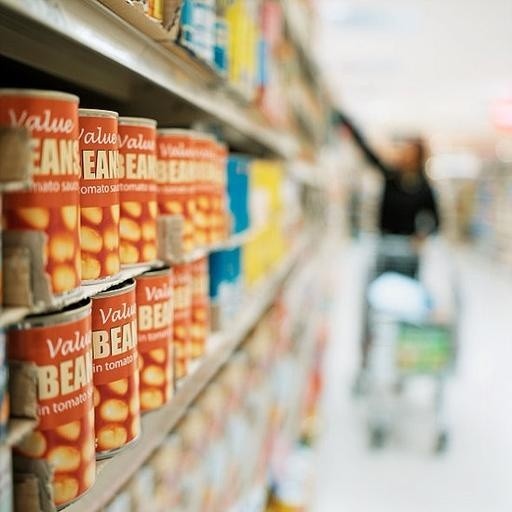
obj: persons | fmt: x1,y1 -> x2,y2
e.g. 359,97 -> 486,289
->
333,107 -> 439,397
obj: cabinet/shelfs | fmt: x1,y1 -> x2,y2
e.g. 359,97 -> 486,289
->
0,9 -> 299,512
